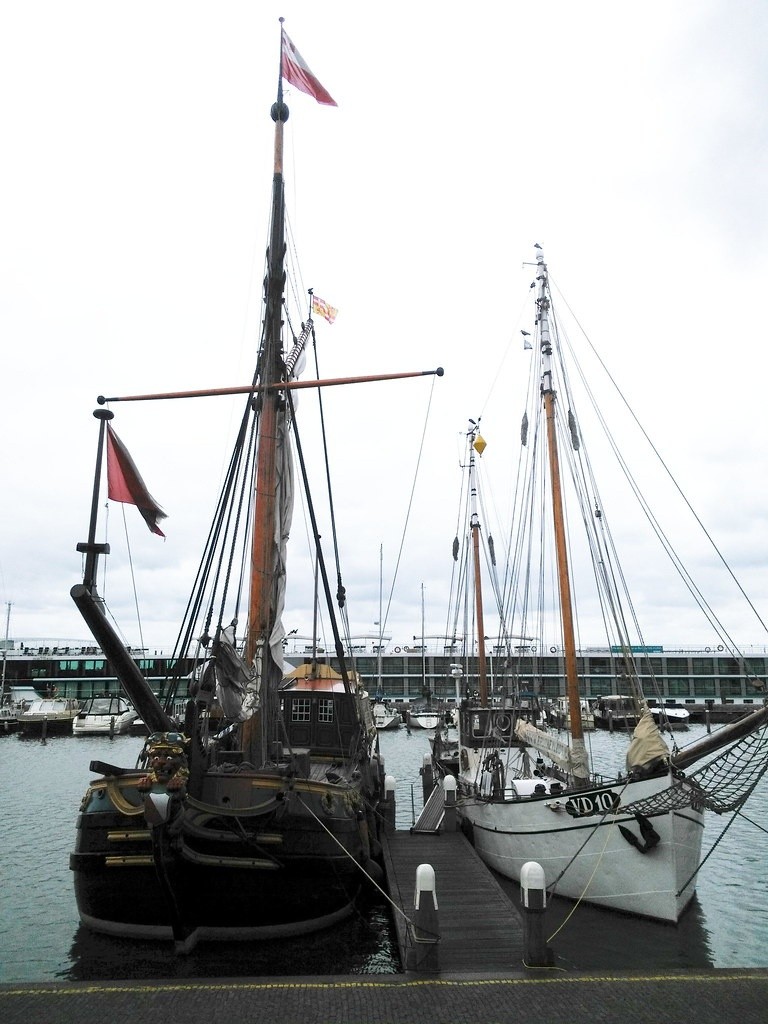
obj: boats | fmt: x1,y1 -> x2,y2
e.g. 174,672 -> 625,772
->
642,704 -> 690,730
0,704 -> 23,735
368,695 -> 403,730
406,581 -> 441,730
71,695 -> 141,735
16,697 -> 85,734
129,716 -> 179,738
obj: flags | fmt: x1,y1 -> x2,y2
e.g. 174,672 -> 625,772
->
313,295 -> 337,324
107,422 -> 169,536
281,26 -> 341,105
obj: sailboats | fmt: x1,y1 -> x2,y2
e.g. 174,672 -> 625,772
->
419,413 -> 553,812
65,17 -> 447,955
551,697 -> 595,732
509,690 -> 548,735
452,238 -> 768,929
590,692 -> 643,733
